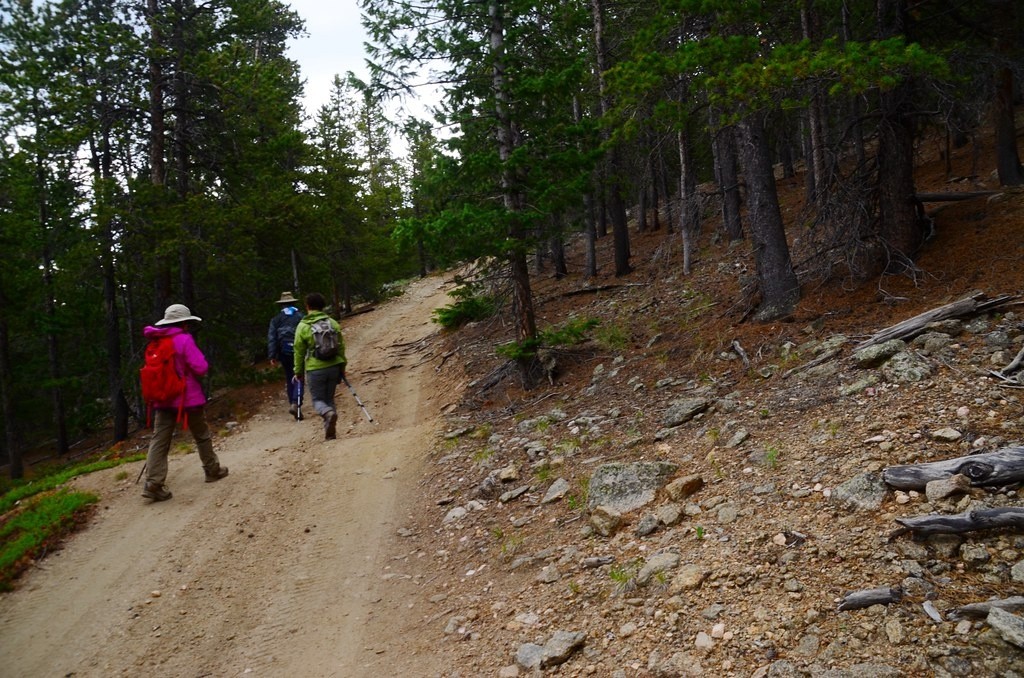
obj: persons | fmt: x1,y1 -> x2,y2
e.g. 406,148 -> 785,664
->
293,293 -> 347,441
141,304 -> 228,500
268,291 -> 305,420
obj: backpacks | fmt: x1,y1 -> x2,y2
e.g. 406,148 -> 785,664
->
277,320 -> 300,356
140,332 -> 188,430
305,318 -> 338,361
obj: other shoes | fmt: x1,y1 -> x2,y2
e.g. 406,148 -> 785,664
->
289,404 -> 304,420
141,482 -> 172,501
323,411 -> 337,439
205,467 -> 228,482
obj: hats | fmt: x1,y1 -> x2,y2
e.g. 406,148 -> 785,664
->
276,292 -> 298,302
155,305 -> 201,325
